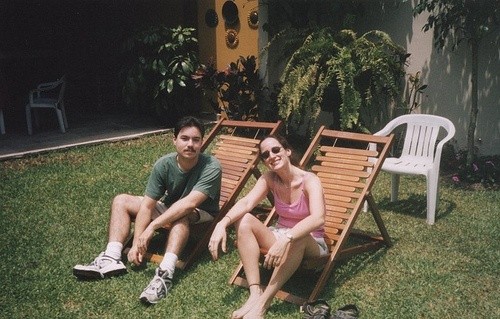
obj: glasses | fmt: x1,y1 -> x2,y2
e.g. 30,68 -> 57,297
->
260,146 -> 283,158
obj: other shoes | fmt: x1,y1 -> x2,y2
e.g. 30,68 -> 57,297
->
331,304 -> 361,319
301,299 -> 331,319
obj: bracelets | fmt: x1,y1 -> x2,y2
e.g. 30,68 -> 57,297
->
224,216 -> 232,225
285,233 -> 293,242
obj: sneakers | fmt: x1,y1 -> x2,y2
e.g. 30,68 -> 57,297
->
73,252 -> 127,279
138,266 -> 173,308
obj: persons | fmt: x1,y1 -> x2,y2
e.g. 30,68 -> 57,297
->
73,116 -> 223,304
209,134 -> 330,319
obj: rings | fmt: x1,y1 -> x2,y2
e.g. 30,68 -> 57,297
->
208,245 -> 211,248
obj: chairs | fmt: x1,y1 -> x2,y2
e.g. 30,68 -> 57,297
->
121,113 -> 456,313
24,73 -> 69,135
